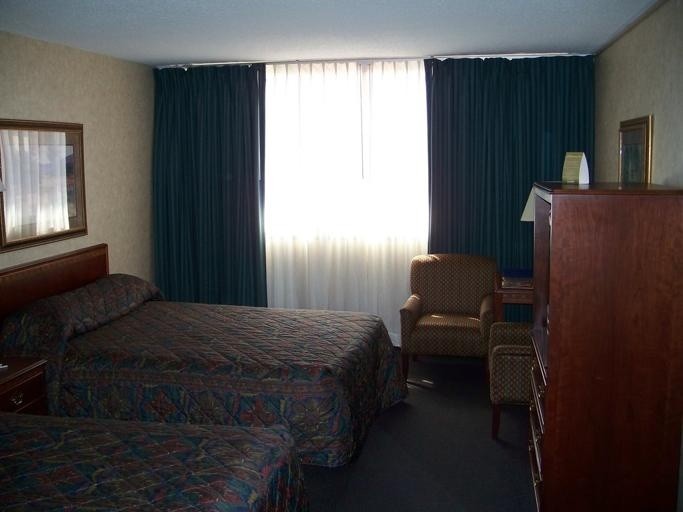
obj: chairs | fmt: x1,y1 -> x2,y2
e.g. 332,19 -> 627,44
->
488,322 -> 532,434
399,254 -> 499,386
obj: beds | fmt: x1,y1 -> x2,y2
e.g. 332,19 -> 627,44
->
0,243 -> 382,468
0,413 -> 296,512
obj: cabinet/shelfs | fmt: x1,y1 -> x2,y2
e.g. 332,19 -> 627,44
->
528,184 -> 683,512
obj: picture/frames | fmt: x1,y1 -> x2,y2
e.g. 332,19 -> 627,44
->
0,119 -> 88,253
618,114 -> 652,186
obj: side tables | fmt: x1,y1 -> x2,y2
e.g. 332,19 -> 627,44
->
494,278 -> 535,322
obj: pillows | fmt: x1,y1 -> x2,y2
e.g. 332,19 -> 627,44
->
28,273 -> 149,338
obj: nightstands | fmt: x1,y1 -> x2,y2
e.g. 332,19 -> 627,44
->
0,357 -> 50,416
